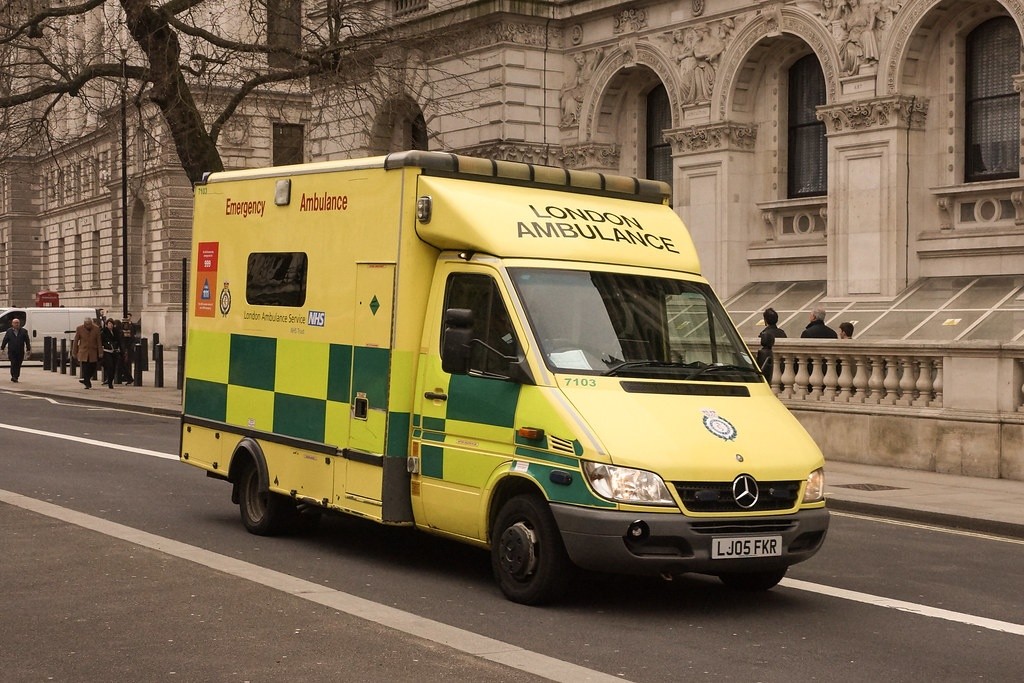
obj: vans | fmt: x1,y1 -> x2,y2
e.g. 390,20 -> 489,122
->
0,308 -> 98,367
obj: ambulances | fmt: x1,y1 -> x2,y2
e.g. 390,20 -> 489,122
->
179,149 -> 831,607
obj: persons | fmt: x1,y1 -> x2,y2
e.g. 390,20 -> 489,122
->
758,308 -> 787,338
839,322 -> 869,385
101,318 -> 124,389
78,318 -> 108,386
99,308 -> 148,384
72,318 -> 104,388
800,307 -> 842,391
0,319 -> 31,382
757,332 -> 785,391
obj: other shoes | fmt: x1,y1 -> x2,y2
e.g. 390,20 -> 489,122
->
11,377 -> 18,382
126,379 -> 134,385
102,380 -> 108,386
79,380 -> 85,383
109,384 -> 113,389
